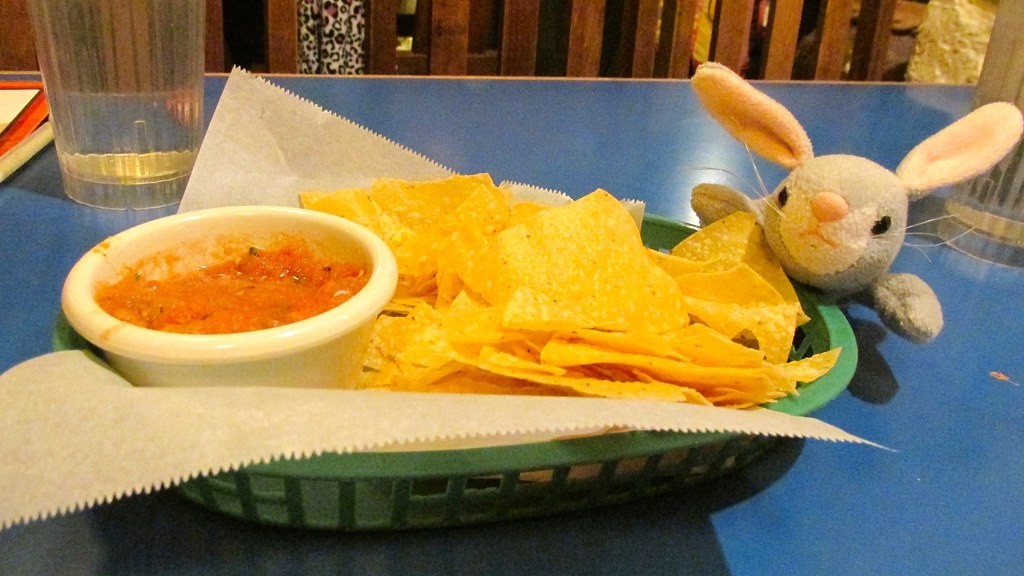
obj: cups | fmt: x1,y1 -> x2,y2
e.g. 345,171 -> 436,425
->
26,0 -> 207,211
937,1 -> 1024,270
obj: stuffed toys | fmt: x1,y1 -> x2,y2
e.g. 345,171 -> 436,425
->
690,63 -> 1023,345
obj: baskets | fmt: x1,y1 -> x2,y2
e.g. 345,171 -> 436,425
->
52,211 -> 858,533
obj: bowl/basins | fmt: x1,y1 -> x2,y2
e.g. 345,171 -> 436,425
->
61,205 -> 398,392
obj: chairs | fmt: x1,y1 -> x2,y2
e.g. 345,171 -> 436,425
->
0,0 -> 896,82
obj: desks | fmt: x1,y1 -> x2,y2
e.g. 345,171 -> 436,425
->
0,70 -> 1024,576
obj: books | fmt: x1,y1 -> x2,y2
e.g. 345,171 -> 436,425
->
0,80 -> 55,183
